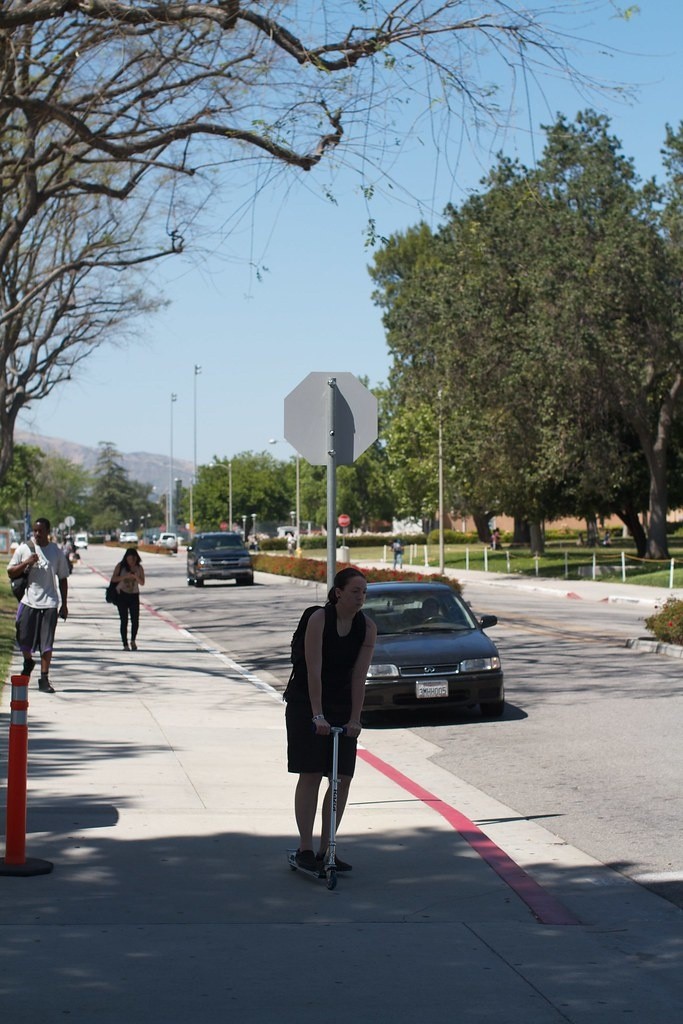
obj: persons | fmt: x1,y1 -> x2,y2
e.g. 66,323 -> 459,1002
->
7,517 -> 69,693
491,526 -> 503,551
34,529 -> 80,574
574,526 -> 613,546
251,535 -> 261,554
283,568 -> 377,872
284,531 -> 297,557
391,537 -> 404,569
105,548 -> 144,651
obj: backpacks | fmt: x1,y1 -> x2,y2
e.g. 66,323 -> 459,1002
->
291,606 -> 322,681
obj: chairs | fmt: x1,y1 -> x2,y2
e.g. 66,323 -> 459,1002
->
400,608 -> 424,625
363,609 -> 392,633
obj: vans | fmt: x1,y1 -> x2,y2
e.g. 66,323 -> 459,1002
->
73,532 -> 88,548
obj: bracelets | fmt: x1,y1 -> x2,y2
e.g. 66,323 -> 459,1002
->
349,719 -> 363,728
311,715 -> 324,721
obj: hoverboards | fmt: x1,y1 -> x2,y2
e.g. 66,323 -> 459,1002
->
288,719 -> 352,891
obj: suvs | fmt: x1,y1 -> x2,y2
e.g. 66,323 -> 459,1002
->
155,533 -> 178,553
186,532 -> 254,586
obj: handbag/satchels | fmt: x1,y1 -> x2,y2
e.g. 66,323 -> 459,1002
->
11,571 -> 28,602
106,581 -> 119,603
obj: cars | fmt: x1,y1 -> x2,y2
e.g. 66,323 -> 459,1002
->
120,532 -> 138,543
348,580 -> 505,729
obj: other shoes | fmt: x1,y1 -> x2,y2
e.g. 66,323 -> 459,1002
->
130,641 -> 137,650
124,645 -> 130,651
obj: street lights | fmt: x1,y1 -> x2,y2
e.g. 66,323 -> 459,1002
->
251,514 -> 257,534
290,511 -> 296,525
242,515 -> 247,541
169,393 -> 177,531
270,438 -> 299,557
175,476 -> 194,541
208,462 -> 233,532
193,365 -> 201,482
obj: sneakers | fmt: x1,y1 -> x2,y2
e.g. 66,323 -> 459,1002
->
38,679 -> 54,693
316,852 -> 352,872
295,848 -> 321,872
21,658 -> 35,677
